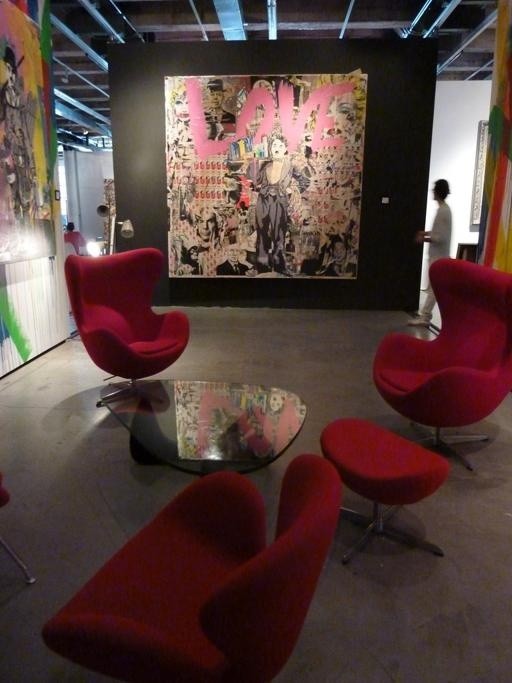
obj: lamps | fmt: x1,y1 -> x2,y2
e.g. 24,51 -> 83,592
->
96,204 -> 134,256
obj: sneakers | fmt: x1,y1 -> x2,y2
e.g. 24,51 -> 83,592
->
408,316 -> 432,327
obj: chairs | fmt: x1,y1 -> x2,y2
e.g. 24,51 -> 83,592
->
373,260 -> 512,471
40,451 -> 344,682
64,247 -> 189,413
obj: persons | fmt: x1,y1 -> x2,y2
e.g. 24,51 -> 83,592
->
96,204 -> 110,217
13,125 -> 32,221
405,177 -> 452,327
64,221 -> 89,255
1,43 -> 39,223
166,76 -> 360,280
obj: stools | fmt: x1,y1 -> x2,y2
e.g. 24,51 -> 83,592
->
320,415 -> 451,566
